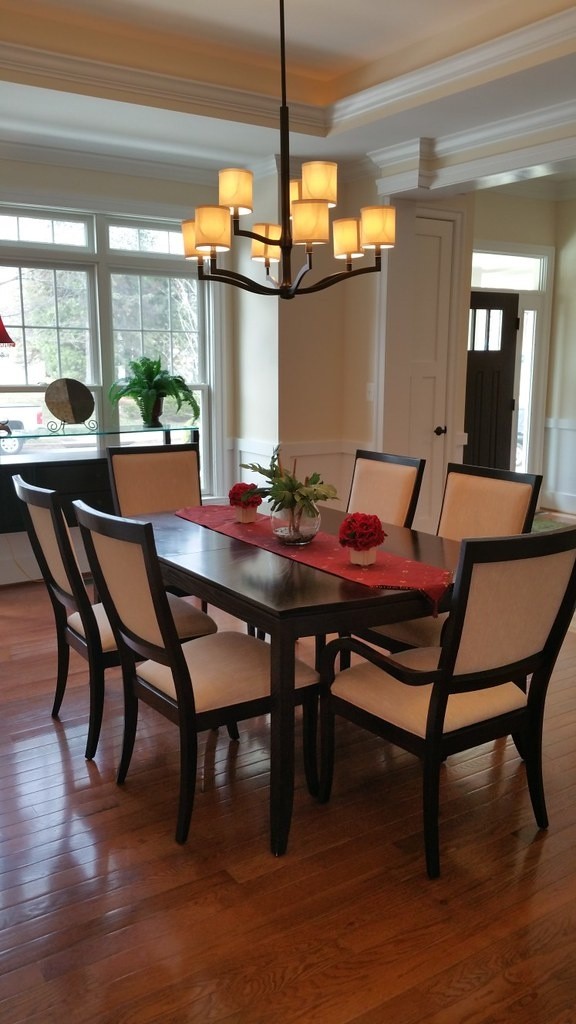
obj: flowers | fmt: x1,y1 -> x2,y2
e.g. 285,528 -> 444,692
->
339,511 -> 387,552
228,482 -> 263,509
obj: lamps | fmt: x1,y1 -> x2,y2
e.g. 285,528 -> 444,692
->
180,0 -> 395,299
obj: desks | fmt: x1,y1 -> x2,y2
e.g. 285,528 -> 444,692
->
133,503 -> 463,855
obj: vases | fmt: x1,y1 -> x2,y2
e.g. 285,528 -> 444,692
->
351,547 -> 378,565
235,506 -> 257,523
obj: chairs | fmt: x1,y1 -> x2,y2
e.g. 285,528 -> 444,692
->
72,499 -> 320,844
13,474 -> 240,760
315,525 -> 576,877
337,463 -> 542,667
107,443 -> 209,615
258,448 -> 427,669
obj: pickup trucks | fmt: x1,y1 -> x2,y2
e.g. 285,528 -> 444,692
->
0,400 -> 45,455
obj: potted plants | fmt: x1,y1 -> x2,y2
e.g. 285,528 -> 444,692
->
109,356 -> 199,428
237,442 -> 338,544
